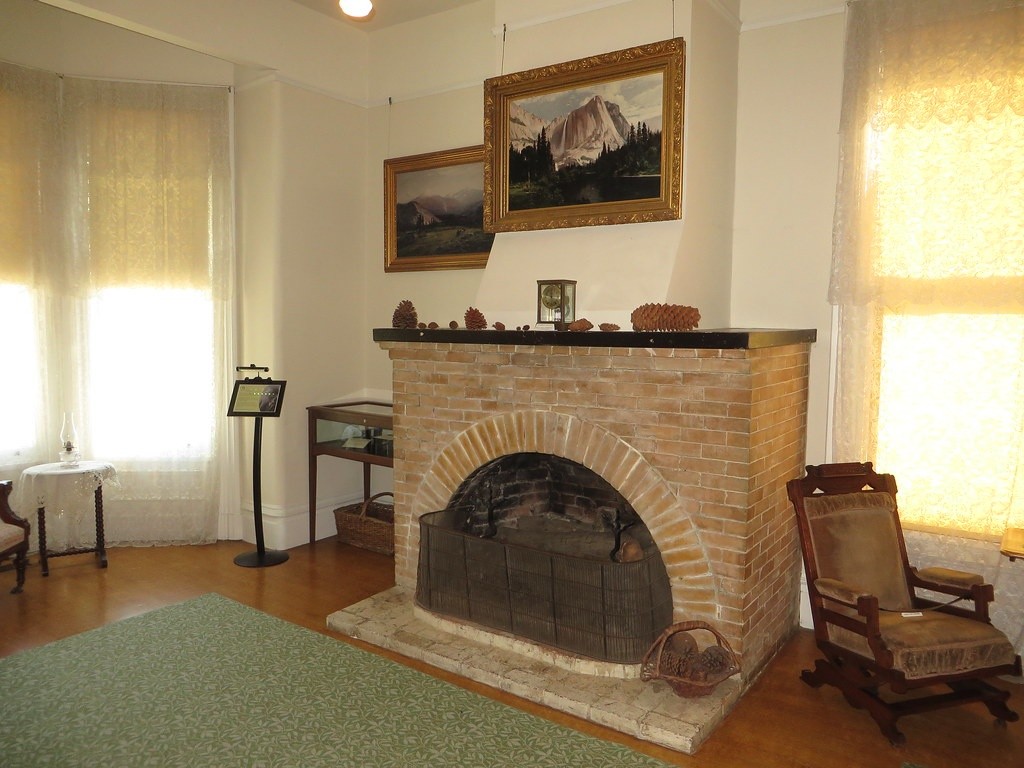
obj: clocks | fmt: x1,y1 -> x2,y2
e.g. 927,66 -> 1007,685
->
537,279 -> 578,330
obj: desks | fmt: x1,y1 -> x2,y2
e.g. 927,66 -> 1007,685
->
15,460 -> 121,577
306,401 -> 392,548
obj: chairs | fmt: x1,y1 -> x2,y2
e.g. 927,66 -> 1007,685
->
0,480 -> 30,586
787,460 -> 1024,749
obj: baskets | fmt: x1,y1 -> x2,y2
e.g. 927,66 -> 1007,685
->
333,491 -> 395,558
639,620 -> 742,698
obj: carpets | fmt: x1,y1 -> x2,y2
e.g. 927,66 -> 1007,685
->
0,592 -> 674,768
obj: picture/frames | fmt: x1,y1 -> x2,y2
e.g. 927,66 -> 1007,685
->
383,143 -> 495,273
483,36 -> 687,233
